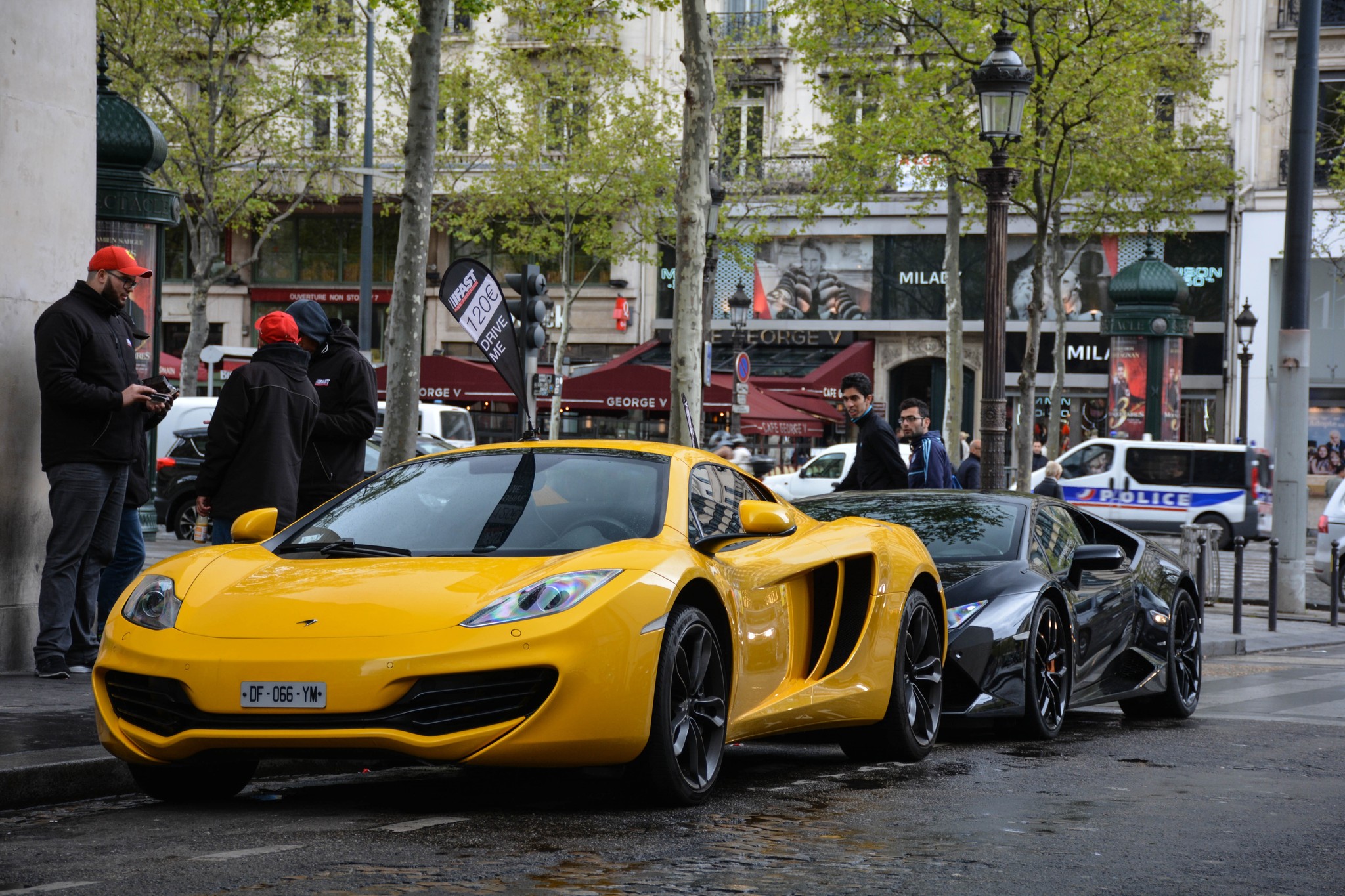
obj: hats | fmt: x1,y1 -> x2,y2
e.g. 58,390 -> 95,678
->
254,311 -> 302,345
87,245 -> 153,278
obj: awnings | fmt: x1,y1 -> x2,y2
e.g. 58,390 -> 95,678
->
364,340 -> 876,469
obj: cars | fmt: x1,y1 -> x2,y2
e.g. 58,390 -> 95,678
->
367,430 -> 460,460
152,427 -> 381,541
758,440 -> 912,501
1312,476 -> 1345,600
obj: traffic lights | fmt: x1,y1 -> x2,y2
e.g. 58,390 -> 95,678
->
503,263 -> 541,346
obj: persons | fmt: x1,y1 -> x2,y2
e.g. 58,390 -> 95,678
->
94,300 -> 379,642
33,244 -> 172,680
766,239 -> 865,318
1308,428 -> 1345,477
708,373 -> 1068,503
1011,241 -> 1182,418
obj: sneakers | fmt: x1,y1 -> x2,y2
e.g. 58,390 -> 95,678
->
65,653 -> 97,674
34,656 -> 70,678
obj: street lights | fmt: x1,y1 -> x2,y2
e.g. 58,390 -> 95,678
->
698,161 -> 731,450
1233,296 -> 1261,448
971,2 -> 1035,495
726,278 -> 753,441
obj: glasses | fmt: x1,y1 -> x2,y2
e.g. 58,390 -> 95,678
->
97,270 -> 138,290
898,417 -> 925,423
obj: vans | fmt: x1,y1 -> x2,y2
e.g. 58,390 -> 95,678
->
1007,433 -> 1272,549
153,394 -> 219,463
370,403 -> 478,456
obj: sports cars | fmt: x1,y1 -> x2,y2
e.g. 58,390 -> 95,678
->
91,439 -> 949,801
781,488 -> 1206,746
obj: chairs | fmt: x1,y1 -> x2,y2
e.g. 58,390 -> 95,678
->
435,475 -> 554,553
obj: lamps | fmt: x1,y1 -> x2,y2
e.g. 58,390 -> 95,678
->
609,279 -> 629,289
724,312 -> 728,318
224,274 -> 242,284
755,312 -> 760,318
1092,314 -> 1096,321
1066,314 -> 1069,321
242,325 -> 249,335
426,271 -> 441,284
